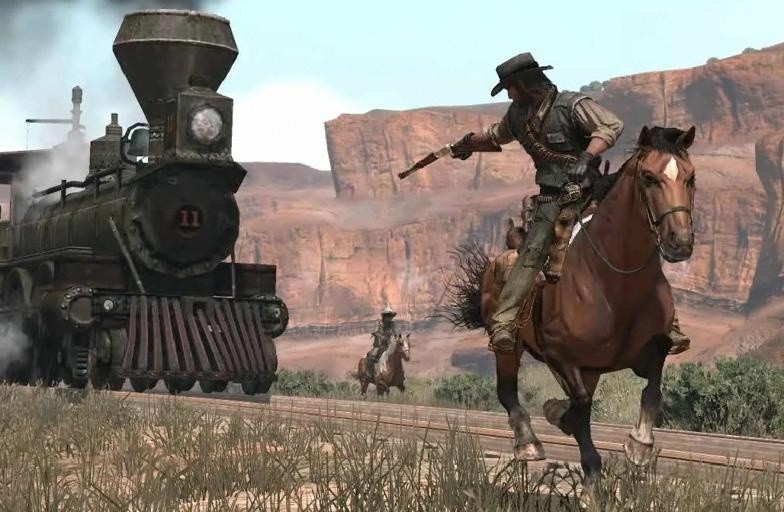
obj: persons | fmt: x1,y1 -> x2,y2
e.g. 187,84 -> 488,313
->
363,305 -> 396,382
449,51 -> 691,355
